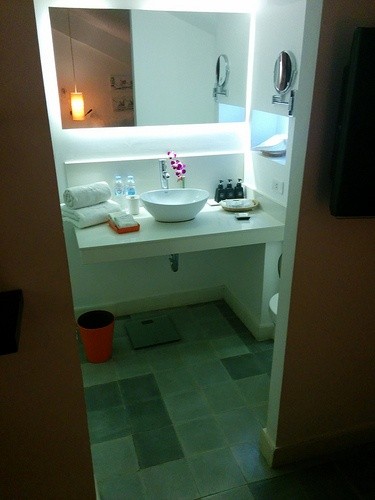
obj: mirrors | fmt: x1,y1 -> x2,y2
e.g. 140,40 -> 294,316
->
48,6 -> 251,130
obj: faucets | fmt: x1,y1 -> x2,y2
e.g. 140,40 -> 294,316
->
158,158 -> 171,189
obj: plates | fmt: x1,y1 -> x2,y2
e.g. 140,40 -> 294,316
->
260,150 -> 285,156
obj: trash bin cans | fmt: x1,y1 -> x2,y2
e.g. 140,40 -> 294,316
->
77,310 -> 115,363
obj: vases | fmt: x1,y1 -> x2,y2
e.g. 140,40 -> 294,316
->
176,176 -> 186,189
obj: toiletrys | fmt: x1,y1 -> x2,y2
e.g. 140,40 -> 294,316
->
234,178 -> 244,198
225,179 -> 235,199
215,180 -> 226,203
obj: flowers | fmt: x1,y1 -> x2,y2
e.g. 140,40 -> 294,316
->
167,151 -> 188,188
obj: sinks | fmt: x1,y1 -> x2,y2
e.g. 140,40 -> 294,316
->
138,188 -> 210,223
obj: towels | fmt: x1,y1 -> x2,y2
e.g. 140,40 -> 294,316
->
60,200 -> 120,229
108,210 -> 137,229
61,181 -> 113,208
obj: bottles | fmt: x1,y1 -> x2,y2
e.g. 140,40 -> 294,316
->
125,175 -> 138,195
113,176 -> 127,196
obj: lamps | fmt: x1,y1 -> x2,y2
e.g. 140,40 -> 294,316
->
67,9 -> 85,120
